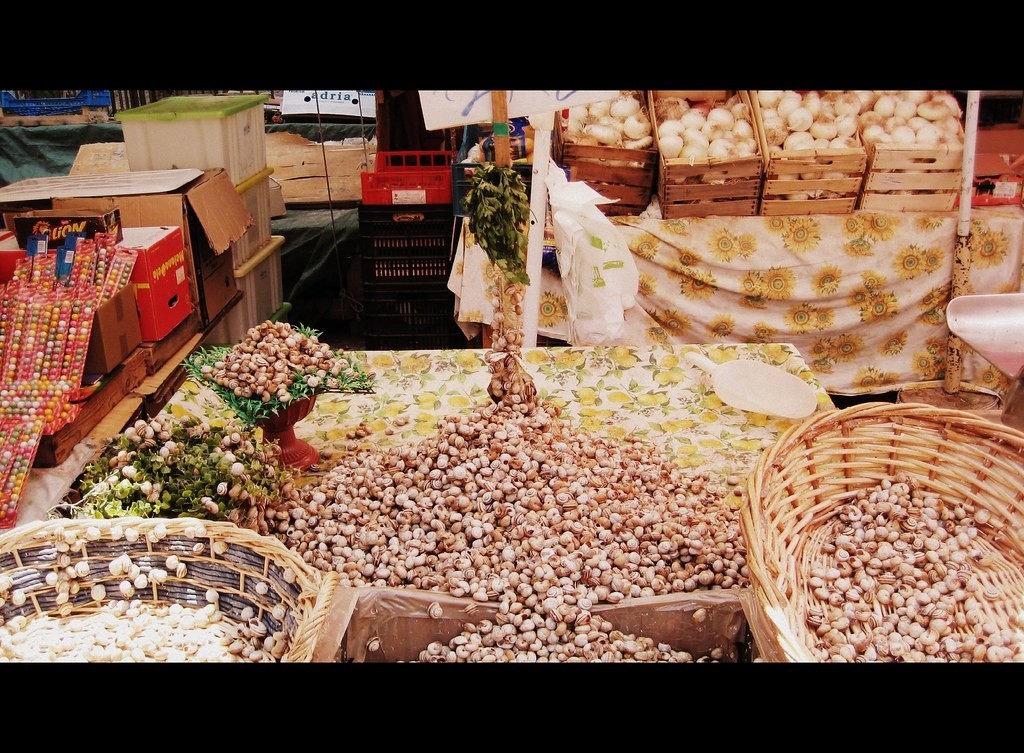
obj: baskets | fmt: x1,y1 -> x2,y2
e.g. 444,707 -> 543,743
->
852,89 -> 964,212
450,123 -> 533,217
737,401 -> 1022,661
553,90 -> 658,217
353,148 -> 465,351
746,87 -> 867,212
1,514 -> 341,663
645,89 -> 760,217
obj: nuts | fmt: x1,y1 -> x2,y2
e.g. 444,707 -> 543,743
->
0,285 -> 1022,663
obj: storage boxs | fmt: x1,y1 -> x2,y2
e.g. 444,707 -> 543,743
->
0,84 -> 1024,666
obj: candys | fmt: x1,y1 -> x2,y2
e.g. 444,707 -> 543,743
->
0,236 -> 134,523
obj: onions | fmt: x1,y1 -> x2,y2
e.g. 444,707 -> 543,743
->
562,90 -> 964,213
